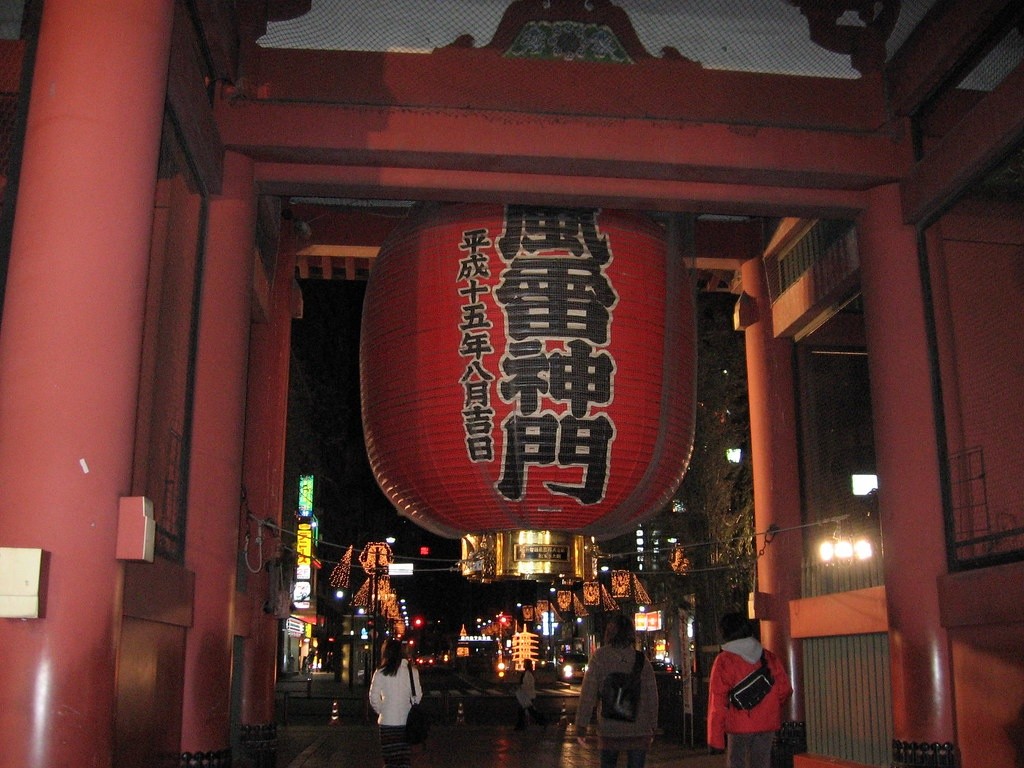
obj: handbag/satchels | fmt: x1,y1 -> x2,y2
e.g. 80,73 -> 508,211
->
404,703 -> 429,744
600,650 -> 645,723
728,648 -> 775,710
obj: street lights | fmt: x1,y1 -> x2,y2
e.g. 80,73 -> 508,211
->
337,588 -> 364,689
547,586 -> 557,661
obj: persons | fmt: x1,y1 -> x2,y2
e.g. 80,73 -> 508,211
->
513,659 -> 549,731
706,613 -> 792,768
369,636 -> 423,768
575,616 -> 658,768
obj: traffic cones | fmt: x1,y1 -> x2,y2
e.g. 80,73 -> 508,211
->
559,700 -> 569,731
454,701 -> 468,728
326,698 -> 343,727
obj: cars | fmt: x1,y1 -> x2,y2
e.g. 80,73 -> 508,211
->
650,660 -> 682,672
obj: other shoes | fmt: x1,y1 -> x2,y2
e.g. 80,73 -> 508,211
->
512,730 -> 528,735
544,721 -> 549,730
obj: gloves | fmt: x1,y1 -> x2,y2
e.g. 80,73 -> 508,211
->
708,745 -> 722,755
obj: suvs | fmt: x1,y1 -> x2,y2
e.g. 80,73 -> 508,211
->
556,649 -> 590,681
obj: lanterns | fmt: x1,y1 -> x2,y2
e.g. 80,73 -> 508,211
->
358,200 -> 699,546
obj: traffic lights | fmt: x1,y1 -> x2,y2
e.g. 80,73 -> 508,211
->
420,546 -> 430,558
500,617 -> 507,623
414,616 -> 423,627
358,627 -> 369,641
